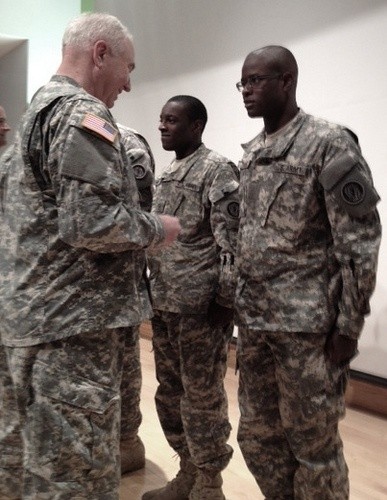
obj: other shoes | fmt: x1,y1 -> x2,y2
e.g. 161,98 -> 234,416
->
120,435 -> 145,475
142,473 -> 195,500
188,475 -> 226,500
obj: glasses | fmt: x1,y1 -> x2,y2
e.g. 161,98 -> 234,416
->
236,76 -> 284,92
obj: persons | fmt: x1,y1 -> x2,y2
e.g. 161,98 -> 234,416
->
0,11 -> 181,500
143,94 -> 241,500
234,44 -> 382,500
